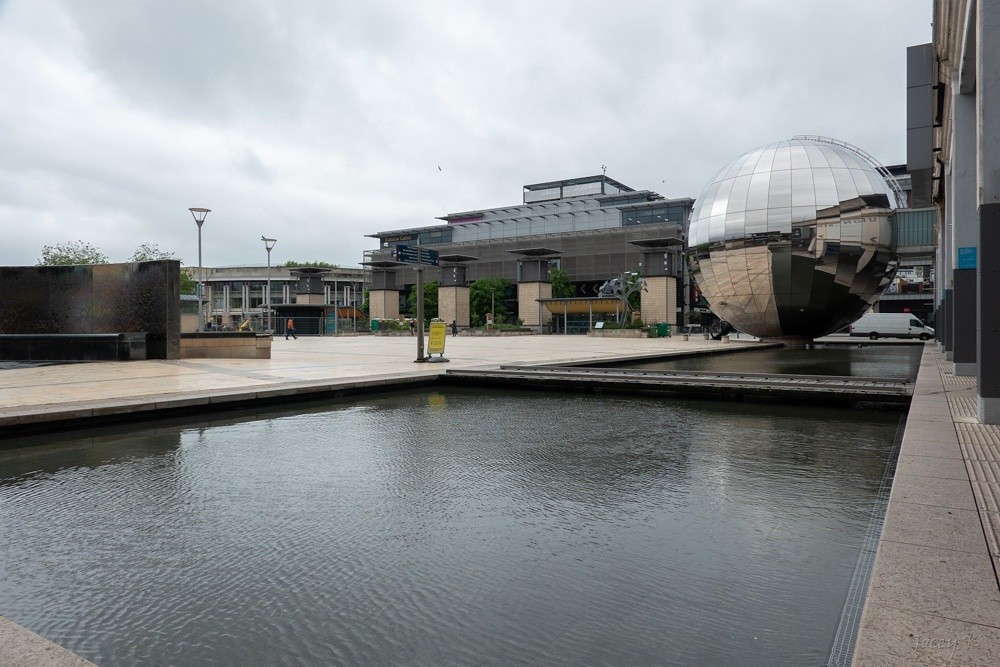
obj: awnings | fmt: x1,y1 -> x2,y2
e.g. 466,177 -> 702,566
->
535,296 -> 636,335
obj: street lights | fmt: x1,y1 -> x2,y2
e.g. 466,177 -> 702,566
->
189,207 -> 212,333
260,238 -> 278,334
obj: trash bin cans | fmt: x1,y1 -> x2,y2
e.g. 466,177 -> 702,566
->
658,323 -> 671,338
371,320 -> 379,330
650,327 -> 658,338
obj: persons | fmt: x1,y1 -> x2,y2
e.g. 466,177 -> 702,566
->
449,320 -> 457,337
286,318 -> 298,340
409,318 -> 415,336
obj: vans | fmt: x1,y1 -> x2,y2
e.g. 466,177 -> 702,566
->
849,312 -> 936,340
